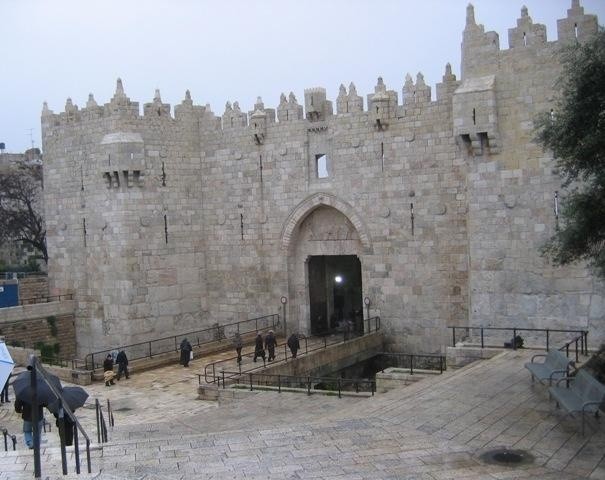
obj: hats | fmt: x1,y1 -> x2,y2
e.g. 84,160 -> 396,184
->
269,330 -> 274,334
107,354 -> 111,358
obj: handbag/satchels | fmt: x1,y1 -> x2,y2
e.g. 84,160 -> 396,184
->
190,351 -> 193,359
183,346 -> 190,351
257,349 -> 265,357
104,371 -> 114,381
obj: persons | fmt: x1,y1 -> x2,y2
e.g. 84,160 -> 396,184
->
104,354 -> 117,386
15,396 -> 47,449
264,330 -> 278,361
116,350 -> 131,380
53,409 -> 74,445
1,372 -> 13,403
288,333 -> 298,358
233,333 -> 242,364
252,330 -> 265,362
179,337 -> 191,369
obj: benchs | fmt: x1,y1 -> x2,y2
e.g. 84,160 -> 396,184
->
524,346 -> 604,436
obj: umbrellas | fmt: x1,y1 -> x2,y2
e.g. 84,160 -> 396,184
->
12,371 -> 62,406
0,339 -> 16,398
59,386 -> 89,411
